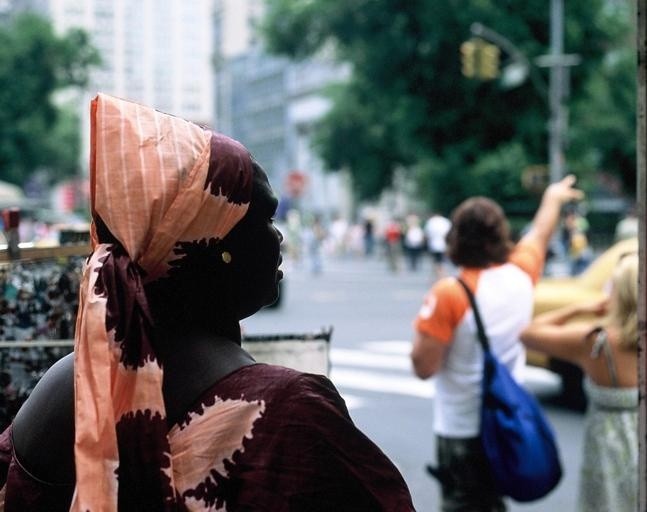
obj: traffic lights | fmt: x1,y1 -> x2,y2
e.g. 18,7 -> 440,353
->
461,38 -> 499,82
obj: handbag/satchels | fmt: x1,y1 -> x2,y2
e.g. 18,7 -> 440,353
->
480,351 -> 563,502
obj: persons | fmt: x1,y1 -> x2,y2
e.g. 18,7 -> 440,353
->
280,173 -> 452,277
27,169 -> 88,239
411,175 -> 584,512
566,208 -> 591,274
615,205 -> 639,240
1,94 -> 416,512
521,252 -> 639,512
